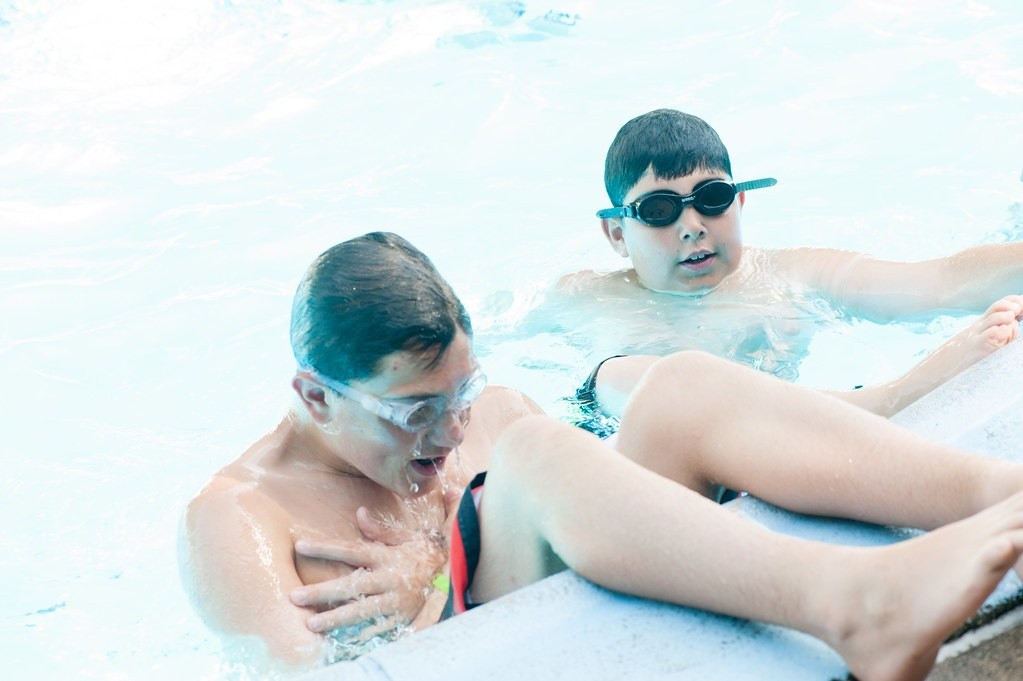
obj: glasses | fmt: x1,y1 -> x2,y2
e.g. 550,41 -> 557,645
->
597,178 -> 777,228
300,364 -> 488,434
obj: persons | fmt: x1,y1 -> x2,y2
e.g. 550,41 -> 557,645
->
586,109 -> 1023,416
188,232 -> 1023,681
